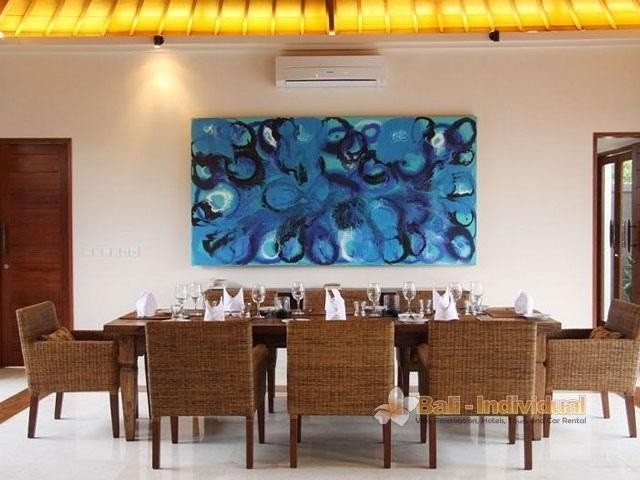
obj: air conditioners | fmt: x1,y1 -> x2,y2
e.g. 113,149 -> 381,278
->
275,55 -> 386,88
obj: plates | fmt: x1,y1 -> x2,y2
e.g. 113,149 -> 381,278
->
523,314 -> 552,320
397,314 -> 424,322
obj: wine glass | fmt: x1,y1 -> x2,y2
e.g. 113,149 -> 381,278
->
450,281 -> 484,316
251,281 -> 266,320
403,283 -> 417,315
368,283 -> 381,318
174,282 -> 203,317
291,281 -> 306,316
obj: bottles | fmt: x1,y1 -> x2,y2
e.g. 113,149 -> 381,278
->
514,289 -> 533,315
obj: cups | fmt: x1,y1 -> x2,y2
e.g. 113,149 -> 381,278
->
274,297 -> 290,320
354,301 -> 367,317
384,294 -> 400,316
420,298 -> 431,315
171,302 -> 183,321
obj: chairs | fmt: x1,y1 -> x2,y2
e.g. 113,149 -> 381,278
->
14,287 -> 639,471
